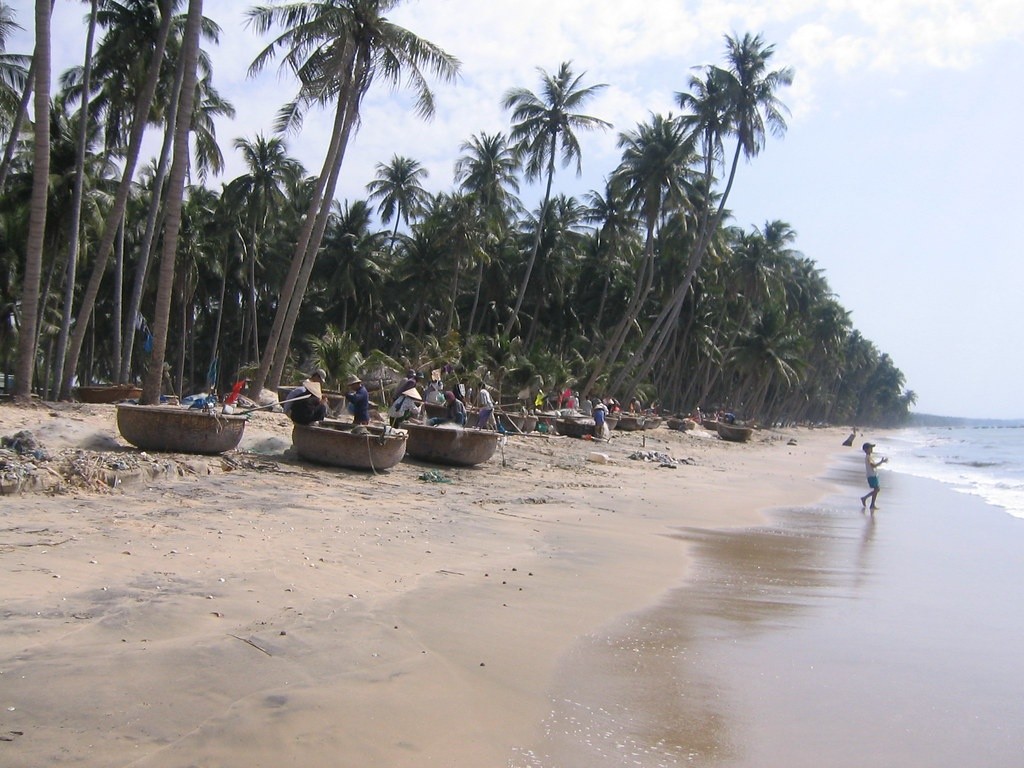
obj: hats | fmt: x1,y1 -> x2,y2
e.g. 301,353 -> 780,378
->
303,381 -> 322,399
594,404 -> 603,409
863,443 -> 875,450
346,375 -> 362,385
408,369 -> 416,376
316,369 -> 328,383
402,387 -> 423,400
416,372 -> 425,379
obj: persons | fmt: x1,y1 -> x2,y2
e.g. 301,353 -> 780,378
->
284,369 -> 370,429
720,411 -> 735,425
632,397 -> 641,413
389,369 -> 494,429
566,391 -> 620,437
861,442 -> 889,510
